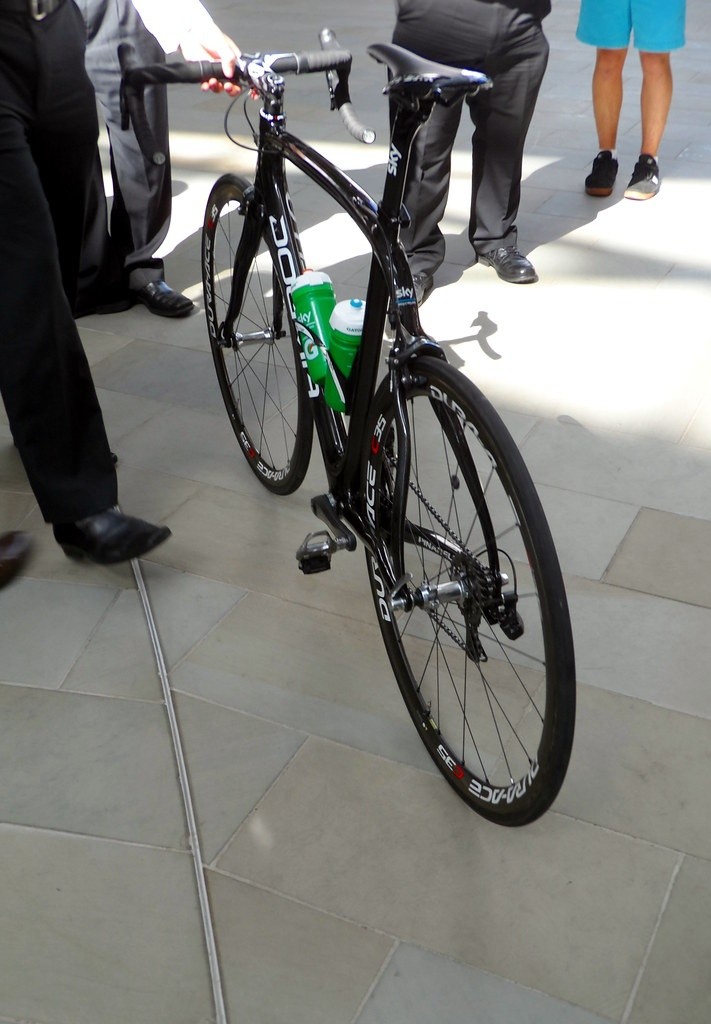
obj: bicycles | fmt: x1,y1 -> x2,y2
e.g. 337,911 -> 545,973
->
115,28 -> 580,827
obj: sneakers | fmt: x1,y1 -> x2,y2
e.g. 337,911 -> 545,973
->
624,154 -> 659,200
585,150 -> 618,196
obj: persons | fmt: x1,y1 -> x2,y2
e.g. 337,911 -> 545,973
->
575,0 -> 686,200
0,0 -> 243,588
388,0 -> 551,308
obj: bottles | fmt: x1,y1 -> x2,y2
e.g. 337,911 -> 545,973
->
324,298 -> 366,412
291,269 -> 336,384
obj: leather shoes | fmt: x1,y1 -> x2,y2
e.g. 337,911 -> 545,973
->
52,509 -> 171,566
70,293 -> 131,319
475,246 -> 536,284
136,280 -> 195,316
411,275 -> 433,303
0,531 -> 31,592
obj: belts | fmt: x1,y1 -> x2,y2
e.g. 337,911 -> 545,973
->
0,0 -> 65,21
